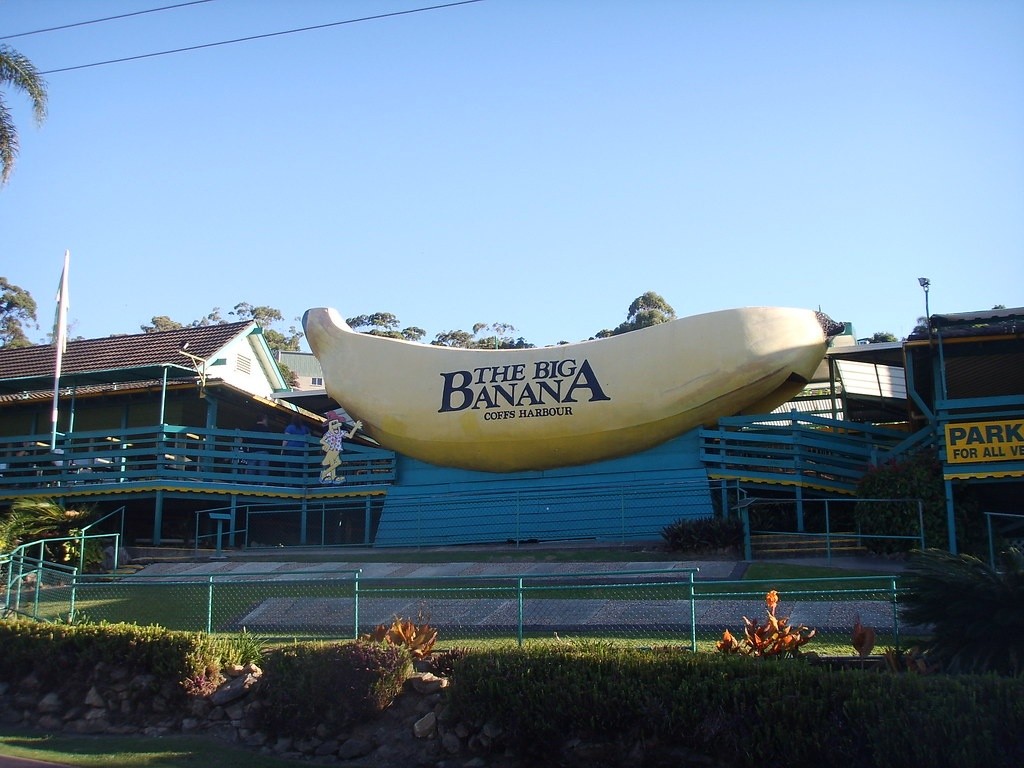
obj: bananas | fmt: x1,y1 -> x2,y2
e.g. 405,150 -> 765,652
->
302,305 -> 844,473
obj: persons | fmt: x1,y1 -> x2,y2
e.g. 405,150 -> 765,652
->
15,441 -> 41,488
242,414 -> 271,486
281,415 -> 311,488
